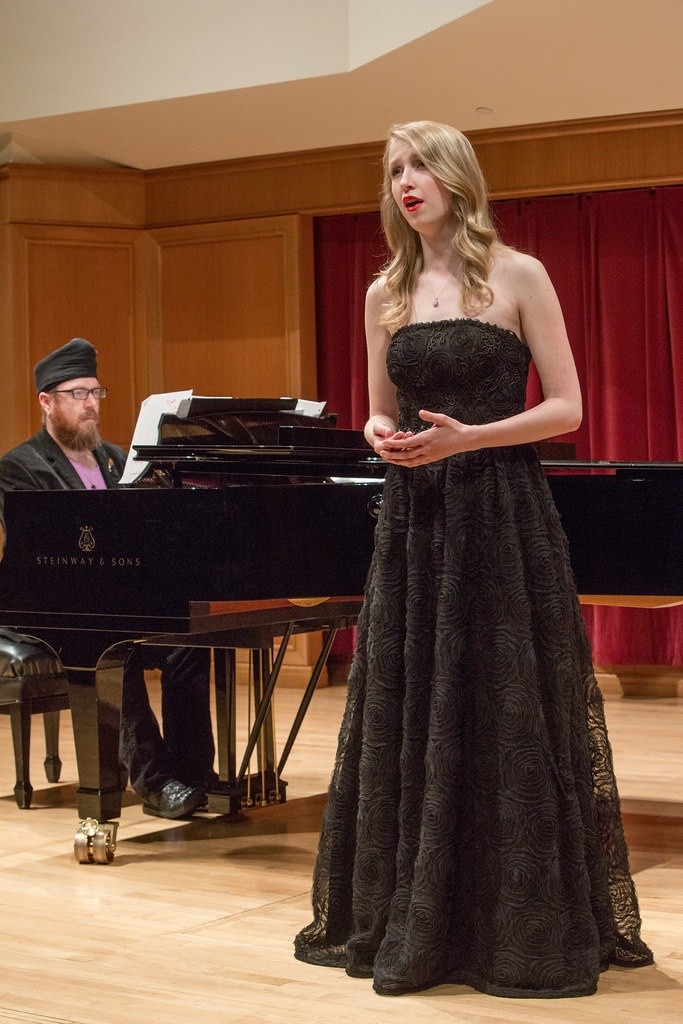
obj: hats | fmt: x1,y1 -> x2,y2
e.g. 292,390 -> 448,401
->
33,338 -> 97,396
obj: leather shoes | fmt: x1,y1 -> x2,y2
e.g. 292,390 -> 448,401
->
142,780 -> 206,819
201,772 -> 228,794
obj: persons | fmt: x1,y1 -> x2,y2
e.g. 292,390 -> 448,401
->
1,337 -> 259,820
291,120 -> 655,999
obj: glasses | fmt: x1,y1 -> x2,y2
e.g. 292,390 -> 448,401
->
47,388 -> 107,400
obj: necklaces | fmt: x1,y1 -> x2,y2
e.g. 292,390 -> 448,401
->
425,260 -> 461,308
77,464 -> 97,489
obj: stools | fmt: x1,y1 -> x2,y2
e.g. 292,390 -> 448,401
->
0,636 -> 72,811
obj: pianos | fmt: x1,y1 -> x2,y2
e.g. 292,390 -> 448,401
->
0,397 -> 683,865
